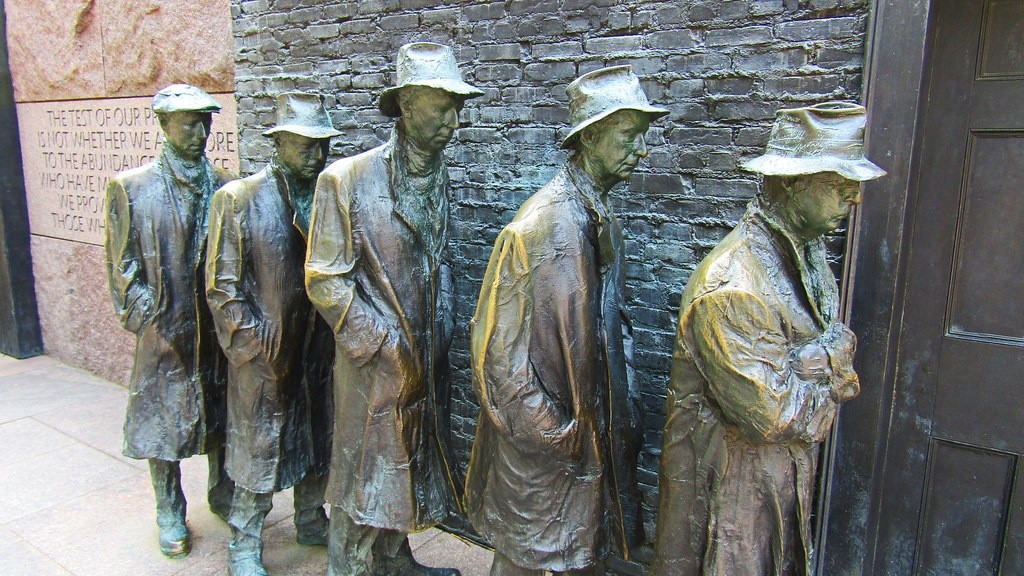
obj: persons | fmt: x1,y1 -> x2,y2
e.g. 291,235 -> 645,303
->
462,64 -> 671,575
205,90 -> 347,575
104,83 -> 241,557
303,40 -> 486,576
649,102 -> 888,576
